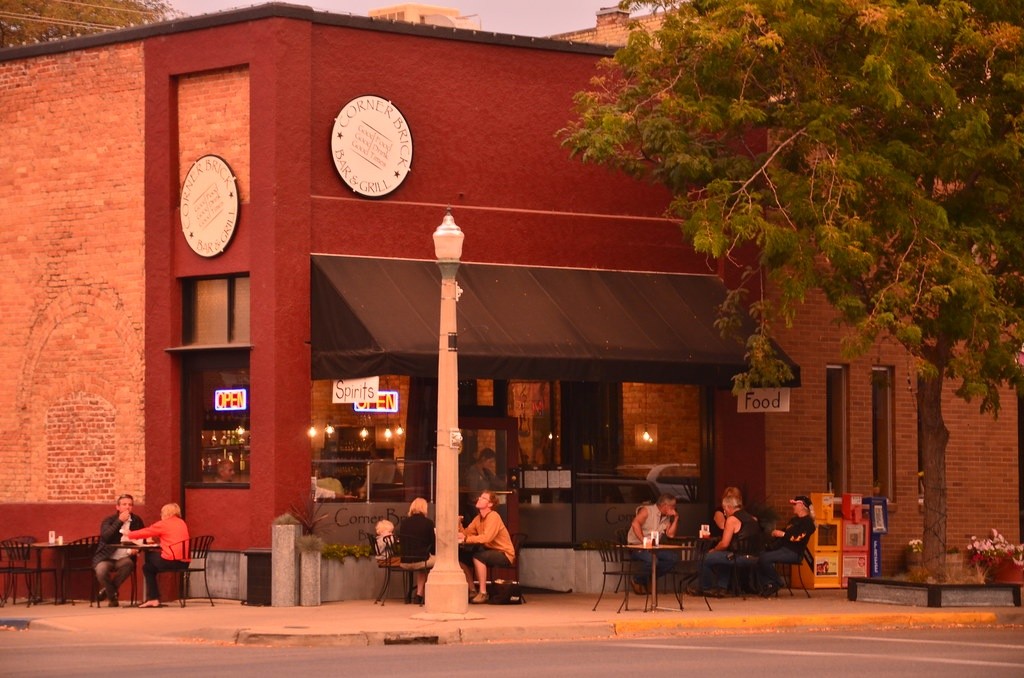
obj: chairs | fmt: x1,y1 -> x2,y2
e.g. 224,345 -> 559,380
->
366,532 -> 529,609
0,535 -> 214,608
592,513 -> 817,612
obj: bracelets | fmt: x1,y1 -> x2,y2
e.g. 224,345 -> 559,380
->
783,532 -> 785,536
464,536 -> 467,542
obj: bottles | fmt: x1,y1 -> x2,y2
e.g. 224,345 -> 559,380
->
207,410 -> 249,423
324,455 -> 367,477
201,432 -> 206,446
228,454 -> 234,462
338,440 -> 375,455
220,430 -> 250,446
211,431 -> 217,447
201,458 -> 221,474
338,477 -> 365,495
239,452 -> 246,472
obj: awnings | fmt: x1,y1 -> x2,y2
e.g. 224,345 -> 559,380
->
311,254 -> 801,387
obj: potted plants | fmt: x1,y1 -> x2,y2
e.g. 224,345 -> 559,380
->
270,514 -> 331,605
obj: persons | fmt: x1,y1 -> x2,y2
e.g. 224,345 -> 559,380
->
374,520 -> 394,560
399,497 -> 436,604
217,459 -> 234,483
359,440 -> 402,494
124,503 -> 191,608
627,494 -> 680,595
459,448 -> 506,517
458,491 -> 515,602
93,494 -> 146,607
758,496 -> 815,595
690,487 -> 764,596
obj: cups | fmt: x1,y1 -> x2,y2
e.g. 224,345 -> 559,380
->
49,531 -> 55,544
147,537 -> 152,542
644,538 -> 652,548
138,539 -> 143,545
701,525 -> 709,534
651,532 -> 659,546
58,536 -> 63,545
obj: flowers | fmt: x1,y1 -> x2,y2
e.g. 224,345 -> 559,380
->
908,540 -> 927,552
967,528 -> 1024,576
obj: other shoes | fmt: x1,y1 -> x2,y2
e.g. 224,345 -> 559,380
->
108,591 -> 119,607
98,588 -> 107,601
413,594 -> 424,604
629,577 -> 647,594
760,582 -> 781,597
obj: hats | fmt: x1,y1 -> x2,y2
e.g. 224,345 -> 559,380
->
790,496 -> 812,511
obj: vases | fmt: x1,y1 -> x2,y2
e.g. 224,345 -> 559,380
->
981,558 -> 1024,586
906,552 -> 923,563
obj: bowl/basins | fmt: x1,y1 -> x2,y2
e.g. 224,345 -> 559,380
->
704,534 -> 709,538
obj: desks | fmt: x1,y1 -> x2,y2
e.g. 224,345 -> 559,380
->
11,540 -> 89,606
611,544 -> 697,612
105,543 -> 159,606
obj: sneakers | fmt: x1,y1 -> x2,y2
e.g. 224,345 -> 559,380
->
469,589 -> 478,599
471,592 -> 489,603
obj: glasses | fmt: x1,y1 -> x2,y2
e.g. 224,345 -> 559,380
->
479,495 -> 490,503
